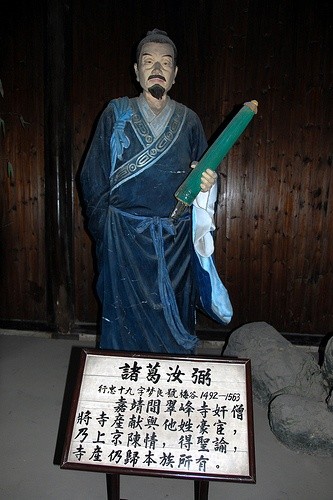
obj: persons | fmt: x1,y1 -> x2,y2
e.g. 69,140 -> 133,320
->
80,28 -> 232,359
